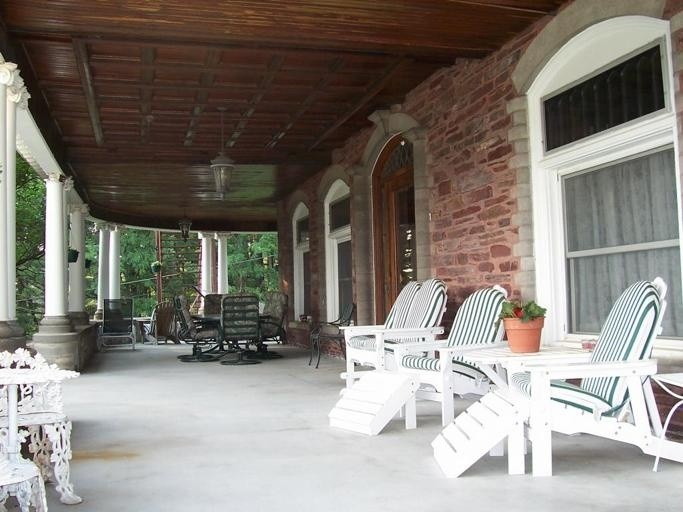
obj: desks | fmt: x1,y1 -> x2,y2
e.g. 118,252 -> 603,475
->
0,369 -> 80,512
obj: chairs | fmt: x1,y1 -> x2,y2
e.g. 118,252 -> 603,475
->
0,347 -> 83,505
0,458 -> 48,512
99,291 -> 289,365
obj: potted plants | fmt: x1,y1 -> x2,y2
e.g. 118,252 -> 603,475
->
151,261 -> 162,272
85,258 -> 92,268
68,245 -> 80,263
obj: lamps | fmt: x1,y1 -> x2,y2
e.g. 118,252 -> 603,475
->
209,107 -> 236,200
178,205 -> 192,244
